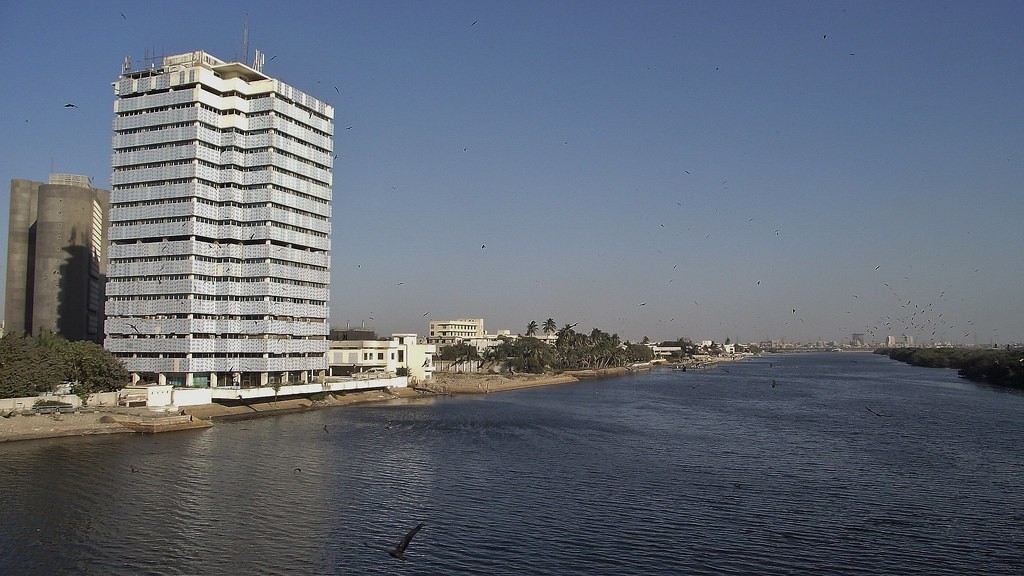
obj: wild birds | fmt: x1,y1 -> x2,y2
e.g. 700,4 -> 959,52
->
64,104 -> 78,108
127,323 -> 139,334
293,468 -> 302,474
687,384 -> 699,390
423,312 -> 428,316
793,309 -> 796,313
639,171 -> 996,347
158,223 -> 404,337
345,127 -> 352,130
323,425 -> 328,433
360,523 -> 426,560
721,369 -> 731,374
866,406 -> 892,417
482,245 -> 486,249
132,468 -> 139,473
179,410 -> 194,422
226,366 -> 234,375
334,155 -> 338,159
